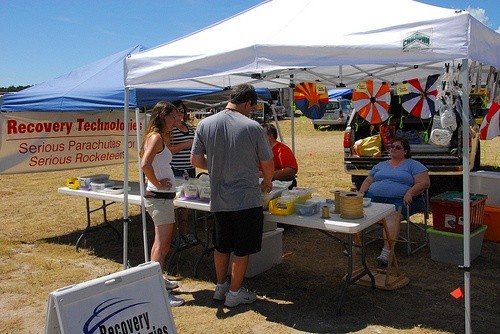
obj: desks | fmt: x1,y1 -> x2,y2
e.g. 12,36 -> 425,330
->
173,195 -> 397,317
57,180 -> 211,279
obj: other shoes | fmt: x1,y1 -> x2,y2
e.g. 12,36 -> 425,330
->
170,231 -> 198,250
375,247 -> 391,265
342,239 -> 364,256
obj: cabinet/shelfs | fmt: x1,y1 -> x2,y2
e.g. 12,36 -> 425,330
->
274,106 -> 286,120
249,101 -> 268,124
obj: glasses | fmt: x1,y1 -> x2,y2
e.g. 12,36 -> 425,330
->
390,143 -> 404,150
177,108 -> 185,113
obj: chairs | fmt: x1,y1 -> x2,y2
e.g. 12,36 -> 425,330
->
357,187 -> 429,258
328,104 -> 333,108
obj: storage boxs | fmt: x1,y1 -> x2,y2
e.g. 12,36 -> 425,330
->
226,228 -> 285,279
429,190 -> 488,234
174,177 -> 212,204
293,199 -> 321,217
425,224 -> 489,266
90,180 -> 116,193
269,191 -> 312,216
65,177 -> 81,190
77,174 -> 111,188
263,221 -> 278,233
409,140 -> 451,155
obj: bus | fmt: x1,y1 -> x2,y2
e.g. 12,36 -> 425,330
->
469,88 -> 486,128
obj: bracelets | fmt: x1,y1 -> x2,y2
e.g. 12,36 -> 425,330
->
158,182 -> 161,190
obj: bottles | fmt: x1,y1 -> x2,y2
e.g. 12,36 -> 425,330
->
182,170 -> 189,181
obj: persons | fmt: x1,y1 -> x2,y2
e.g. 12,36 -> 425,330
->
344,137 -> 430,265
257,122 -> 298,180
191,84 -> 274,305
139,100 -> 184,306
164,99 -> 197,178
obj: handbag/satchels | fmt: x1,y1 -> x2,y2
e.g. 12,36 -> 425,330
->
350,131 -> 382,157
379,120 -> 398,152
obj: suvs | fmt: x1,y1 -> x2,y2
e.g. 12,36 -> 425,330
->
311,98 -> 353,130
343,88 -> 481,190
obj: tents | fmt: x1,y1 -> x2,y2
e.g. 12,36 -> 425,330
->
0,0 -> 500,334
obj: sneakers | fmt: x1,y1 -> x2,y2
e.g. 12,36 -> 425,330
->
223,286 -> 257,306
212,280 -> 230,299
167,292 -> 185,306
162,276 -> 178,289
